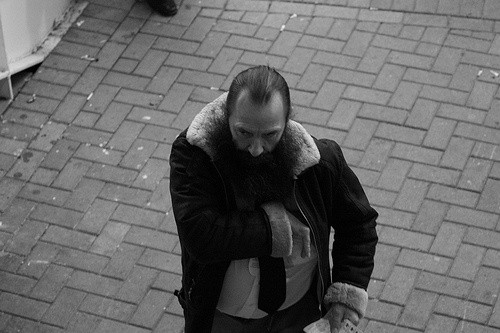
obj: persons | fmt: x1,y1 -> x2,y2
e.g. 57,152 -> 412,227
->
168,65 -> 379,333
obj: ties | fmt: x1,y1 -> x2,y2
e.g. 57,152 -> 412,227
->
258,255 -> 286,317
